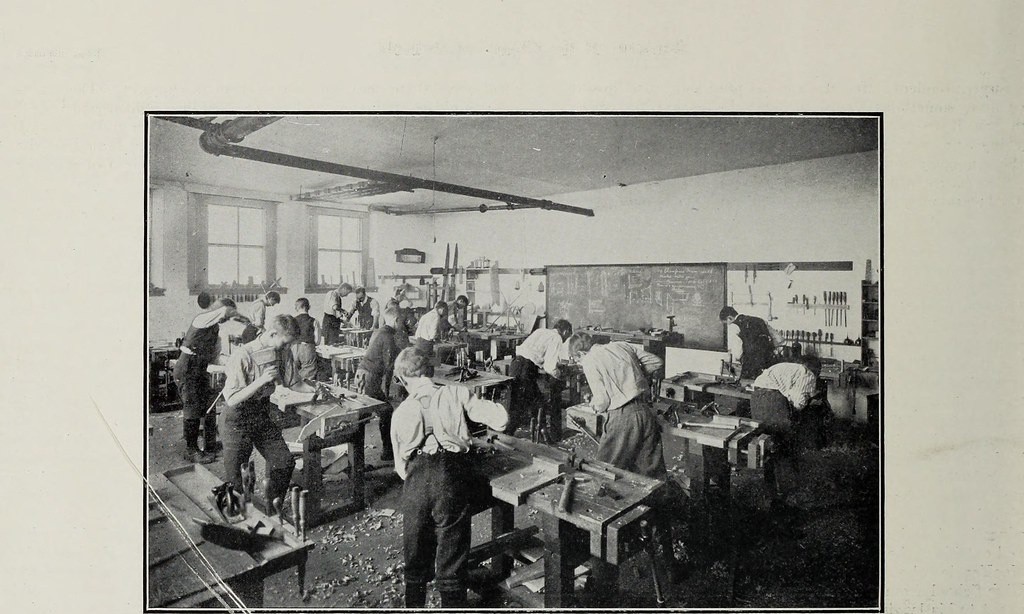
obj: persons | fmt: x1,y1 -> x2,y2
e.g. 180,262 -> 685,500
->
173,283 -> 468,515
390,346 -> 508,608
750,355 -> 822,489
503,319 -> 572,436
569,332 -> 668,481
719,306 -> 775,417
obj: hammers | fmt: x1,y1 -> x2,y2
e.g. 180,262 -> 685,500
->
557,474 -> 576,513
486,432 -> 515,454
248,344 -> 279,387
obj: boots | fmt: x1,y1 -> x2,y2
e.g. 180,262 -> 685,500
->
440,590 -> 468,608
182,418 -> 215,464
265,466 -> 291,524
405,583 -> 426,608
203,417 -> 223,454
379,422 -> 394,461
